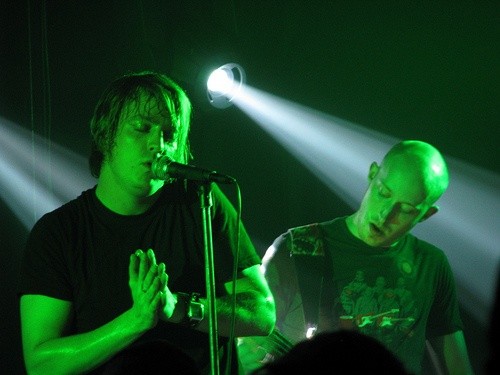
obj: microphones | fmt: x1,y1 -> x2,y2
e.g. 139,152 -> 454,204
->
151,155 -> 237,185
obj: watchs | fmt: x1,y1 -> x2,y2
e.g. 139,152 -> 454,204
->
179,291 -> 204,330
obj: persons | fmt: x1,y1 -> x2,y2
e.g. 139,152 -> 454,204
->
19,71 -> 276,375
236,140 -> 473,375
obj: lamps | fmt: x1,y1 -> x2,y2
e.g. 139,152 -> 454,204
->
172,41 -> 247,113
